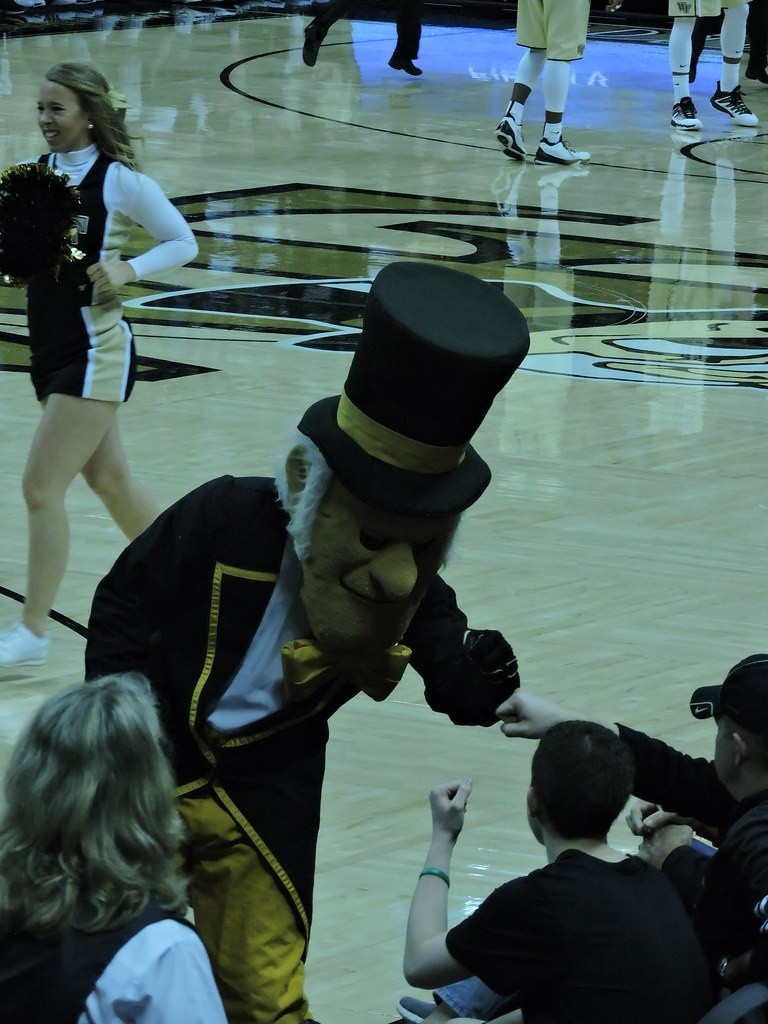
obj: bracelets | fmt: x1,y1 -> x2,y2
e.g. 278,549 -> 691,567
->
418,866 -> 450,891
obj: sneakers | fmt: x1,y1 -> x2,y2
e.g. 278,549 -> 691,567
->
670,126 -> 703,157
0,621 -> 50,667
712,127 -> 758,154
534,165 -> 589,189
670,97 -> 703,130
494,115 -> 528,161
533,135 -> 591,166
489,159 -> 527,209
709,80 -> 759,127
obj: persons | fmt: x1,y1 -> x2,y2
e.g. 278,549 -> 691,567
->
689,0 -> 768,84
388,719 -> 718,1024
301,0 -> 423,76
668,0 -> 759,130
419,653 -> 768,1024
0,670 -> 229,1024
0,62 -> 199,669
494,0 -> 591,165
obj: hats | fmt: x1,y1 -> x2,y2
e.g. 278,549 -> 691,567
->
690,654 -> 768,737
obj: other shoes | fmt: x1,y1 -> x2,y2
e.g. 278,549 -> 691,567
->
745,66 -> 768,84
303,24 -> 326,67
388,53 -> 422,76
397,996 -> 439,1023
688,57 -> 698,83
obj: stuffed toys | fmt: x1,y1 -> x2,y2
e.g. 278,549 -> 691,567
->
82,261 -> 531,1024
